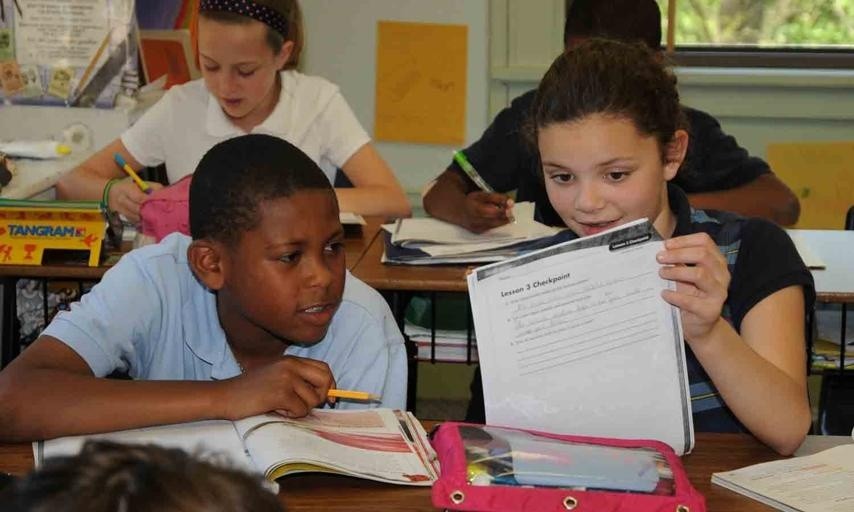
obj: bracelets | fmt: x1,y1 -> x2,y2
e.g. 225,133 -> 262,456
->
99,178 -> 120,210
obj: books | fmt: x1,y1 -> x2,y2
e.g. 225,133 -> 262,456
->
382,197 -> 538,275
707,438 -> 853,511
32,403 -> 440,496
337,212 -> 369,228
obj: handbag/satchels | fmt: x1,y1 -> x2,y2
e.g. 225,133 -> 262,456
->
141,172 -> 192,242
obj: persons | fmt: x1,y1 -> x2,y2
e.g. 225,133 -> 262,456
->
57,3 -> 412,222
0,437 -> 286,512
418,0 -> 800,235
1,132 -> 409,443
465,34 -> 814,460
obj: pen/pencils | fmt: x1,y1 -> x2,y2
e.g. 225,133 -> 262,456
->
115,153 -> 153,195
327,389 -> 381,401
452,149 -> 517,225
464,445 -> 677,496
